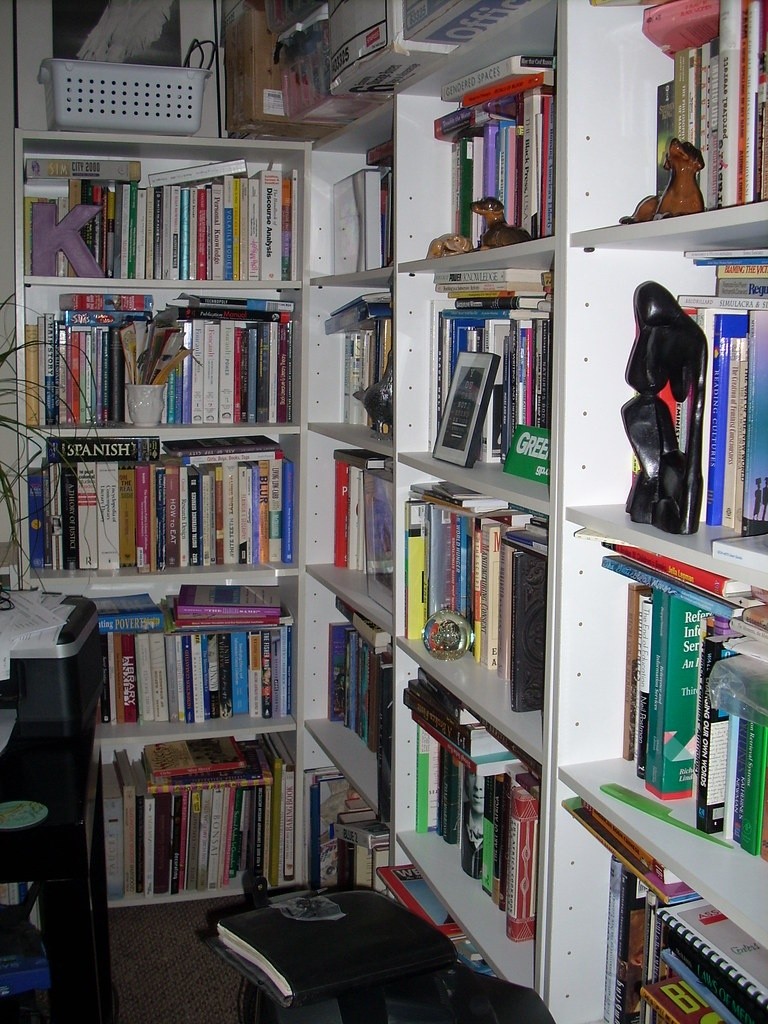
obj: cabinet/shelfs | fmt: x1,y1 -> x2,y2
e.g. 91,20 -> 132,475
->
0,0 -> 768,1024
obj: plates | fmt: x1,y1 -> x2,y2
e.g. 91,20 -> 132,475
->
0,801 -> 48,830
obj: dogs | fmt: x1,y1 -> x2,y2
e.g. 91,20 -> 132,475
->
619,137 -> 705,225
470,196 -> 532,251
426,232 -> 473,261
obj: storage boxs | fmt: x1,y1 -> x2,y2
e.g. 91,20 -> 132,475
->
433,351 -> 500,467
224,11 -> 347,140
277,22 -> 357,120
37,54 -> 212,132
329,0 -> 451,94
301,6 -> 385,121
402,0 -> 531,46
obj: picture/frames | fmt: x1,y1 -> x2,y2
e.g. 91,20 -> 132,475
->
12,0 -> 219,137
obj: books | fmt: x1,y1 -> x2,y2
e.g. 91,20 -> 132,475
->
25,0 -> 768,1024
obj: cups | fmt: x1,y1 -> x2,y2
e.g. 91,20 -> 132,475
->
125,385 -> 166,427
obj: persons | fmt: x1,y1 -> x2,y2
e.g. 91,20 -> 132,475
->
461,770 -> 484,878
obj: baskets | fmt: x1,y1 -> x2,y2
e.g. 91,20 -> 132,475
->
36,58 -> 213,136
